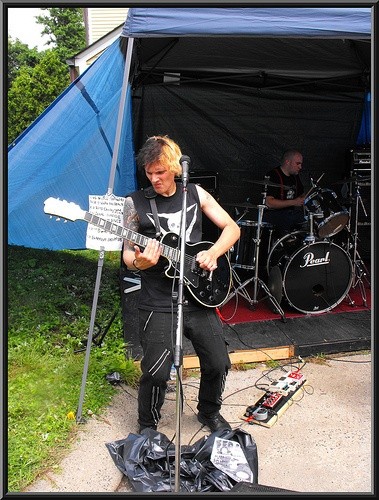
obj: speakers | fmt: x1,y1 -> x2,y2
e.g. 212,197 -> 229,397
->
230,481 -> 298,493
348,167 -> 371,284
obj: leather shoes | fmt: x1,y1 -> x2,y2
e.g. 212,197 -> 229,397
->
198,410 -> 232,433
139,425 -> 157,434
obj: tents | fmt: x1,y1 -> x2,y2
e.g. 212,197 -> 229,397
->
8,8 -> 373,421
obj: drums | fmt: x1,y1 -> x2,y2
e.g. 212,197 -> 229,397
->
266,229 -> 355,315
303,187 -> 350,239
230,219 -> 274,270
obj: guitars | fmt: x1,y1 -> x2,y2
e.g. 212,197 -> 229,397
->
42,196 -> 233,308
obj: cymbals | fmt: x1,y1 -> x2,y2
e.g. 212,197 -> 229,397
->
219,200 -> 275,211
240,176 -> 295,190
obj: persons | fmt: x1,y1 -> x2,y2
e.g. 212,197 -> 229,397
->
122,137 -> 241,437
263,149 -> 306,236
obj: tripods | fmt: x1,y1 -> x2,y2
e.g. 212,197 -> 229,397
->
219,184 -> 288,324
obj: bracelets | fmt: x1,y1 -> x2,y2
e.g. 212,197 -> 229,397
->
133,259 -> 139,271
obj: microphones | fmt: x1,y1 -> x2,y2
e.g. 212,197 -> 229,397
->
179,155 -> 191,188
311,177 -> 322,191
